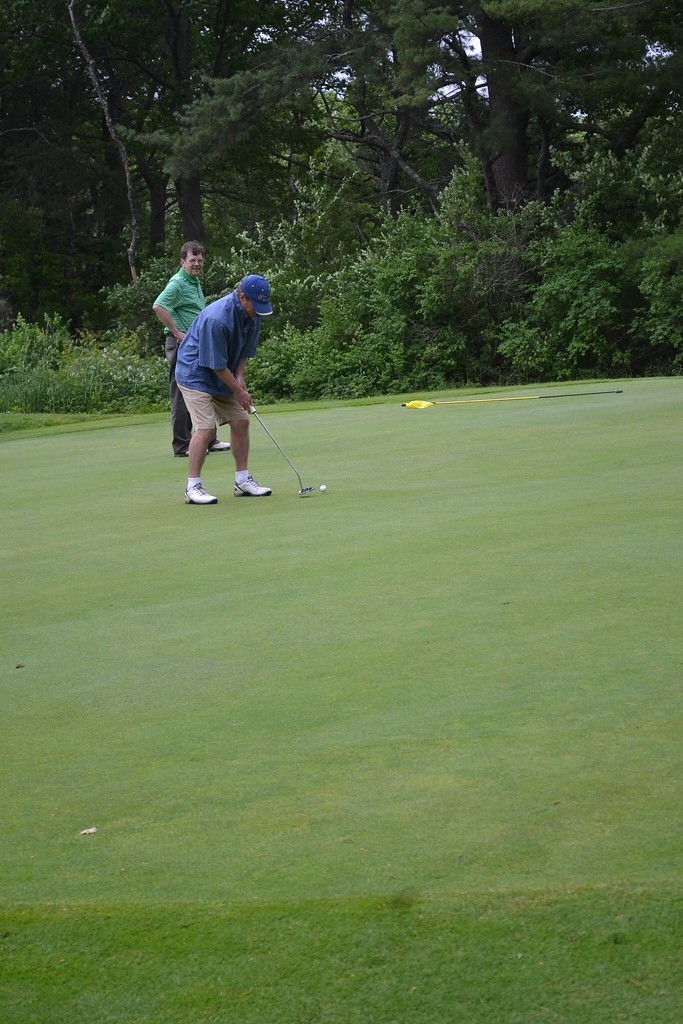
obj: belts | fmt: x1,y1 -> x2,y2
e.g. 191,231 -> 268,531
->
167,333 -> 174,337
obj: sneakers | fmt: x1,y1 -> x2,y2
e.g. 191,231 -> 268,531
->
234,476 -> 272,497
185,448 -> 209,456
209,441 -> 231,451
184,483 -> 218,504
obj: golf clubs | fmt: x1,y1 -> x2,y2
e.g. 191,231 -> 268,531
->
249,403 -> 314,496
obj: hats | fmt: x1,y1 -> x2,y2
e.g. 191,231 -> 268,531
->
240,275 -> 273,315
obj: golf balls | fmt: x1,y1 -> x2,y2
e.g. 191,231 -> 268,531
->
319,484 -> 328,493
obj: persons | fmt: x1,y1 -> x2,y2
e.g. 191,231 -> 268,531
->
153,241 -> 230,457
175,275 -> 272,504
